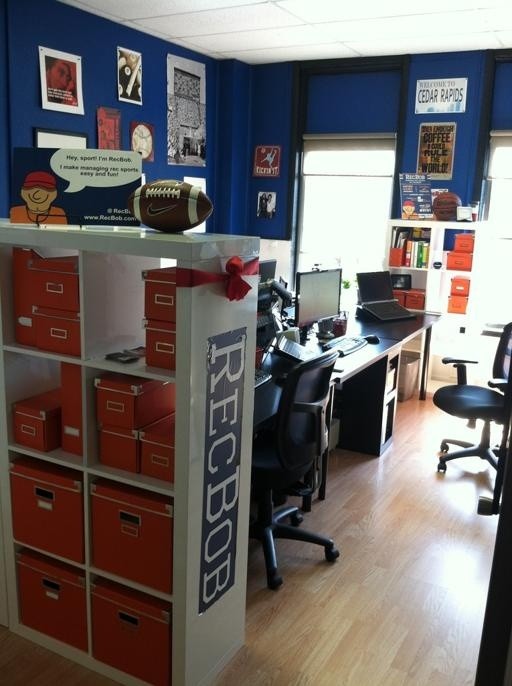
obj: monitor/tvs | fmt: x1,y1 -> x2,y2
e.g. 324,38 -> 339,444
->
295,268 -> 342,339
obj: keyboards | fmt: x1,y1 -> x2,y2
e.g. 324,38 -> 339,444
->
322,335 -> 369,356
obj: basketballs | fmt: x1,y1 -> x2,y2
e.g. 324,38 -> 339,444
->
433,192 -> 462,221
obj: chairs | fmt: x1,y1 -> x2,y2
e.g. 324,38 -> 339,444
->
433,319 -> 512,483
247,345 -> 339,592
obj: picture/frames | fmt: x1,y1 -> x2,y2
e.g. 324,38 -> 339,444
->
32,125 -> 90,150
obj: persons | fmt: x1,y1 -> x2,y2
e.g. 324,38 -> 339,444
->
266,194 -> 276,219
257,193 -> 269,218
45,55 -> 78,106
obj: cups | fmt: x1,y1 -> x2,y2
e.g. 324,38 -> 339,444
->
288,327 -> 301,345
468,200 -> 477,222
334,319 -> 347,335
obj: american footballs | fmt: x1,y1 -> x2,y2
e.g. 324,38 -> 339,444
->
129,181 -> 214,231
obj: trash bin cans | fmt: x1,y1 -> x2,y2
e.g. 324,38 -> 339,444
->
397,350 -> 422,403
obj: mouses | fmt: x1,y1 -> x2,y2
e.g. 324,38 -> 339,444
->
364,335 -> 379,344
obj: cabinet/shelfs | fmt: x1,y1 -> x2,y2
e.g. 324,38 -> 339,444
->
382,214 -> 496,386
0,213 -> 264,684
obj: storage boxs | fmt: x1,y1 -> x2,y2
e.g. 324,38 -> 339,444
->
9,240 -> 180,686
386,231 -> 476,315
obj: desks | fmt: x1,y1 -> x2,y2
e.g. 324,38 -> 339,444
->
337,299 -> 445,403
253,331 -> 403,459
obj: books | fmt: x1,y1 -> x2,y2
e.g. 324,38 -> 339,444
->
391,226 -> 430,269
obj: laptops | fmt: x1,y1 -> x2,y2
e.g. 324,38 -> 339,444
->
356,271 -> 417,322
271,301 -> 314,362
